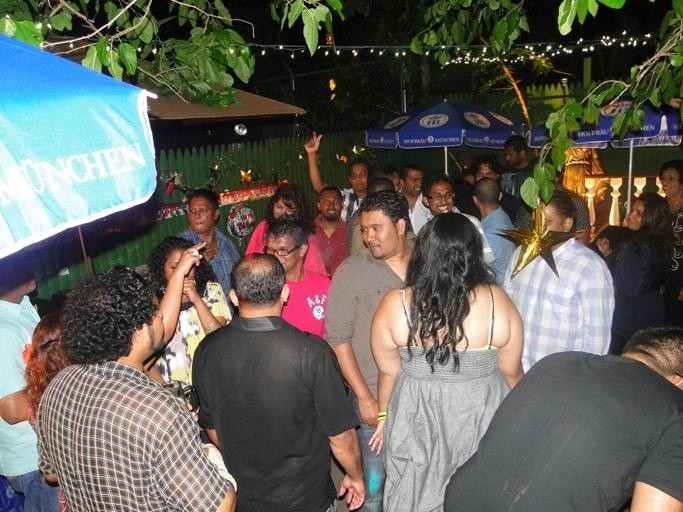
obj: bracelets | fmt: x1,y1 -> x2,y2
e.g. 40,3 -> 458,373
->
376,411 -> 386,422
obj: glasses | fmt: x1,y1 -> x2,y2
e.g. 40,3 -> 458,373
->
263,245 -> 298,256
426,190 -> 455,200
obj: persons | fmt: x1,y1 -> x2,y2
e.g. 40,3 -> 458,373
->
500,189 -> 616,374
180,189 -> 241,316
471,176 -> 516,284
514,158 -> 589,245
412,175 -> 496,266
383,166 -> 404,191
0,279 -> 62,511
463,152 -> 518,222
312,187 -> 350,277
596,193 -> 673,355
245,184 -> 329,277
368,212 -> 524,512
349,177 -> 393,252
398,166 -> 432,234
262,215 -> 330,339
192,251 -> 366,512
35,261 -> 236,511
149,237 -> 232,387
324,191 -> 416,512
26,241 -> 206,511
659,160 -> 683,303
304,131 -> 368,220
499,136 -> 532,216
443,326 -> 683,512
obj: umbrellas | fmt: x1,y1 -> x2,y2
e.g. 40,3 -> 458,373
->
364,97 -> 526,180
527,95 -> 683,225
